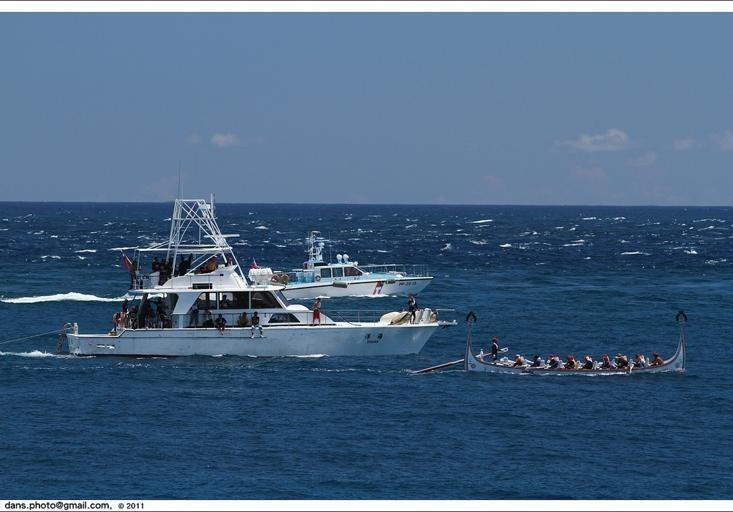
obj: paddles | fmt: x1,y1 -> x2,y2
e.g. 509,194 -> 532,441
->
411,347 -> 508,373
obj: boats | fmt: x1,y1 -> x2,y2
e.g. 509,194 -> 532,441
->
258,230 -> 433,301
462,310 -> 686,376
64,190 -> 458,359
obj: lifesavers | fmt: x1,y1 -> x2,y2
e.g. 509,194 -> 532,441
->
281,275 -> 288,282
315,276 -> 321,281
271,274 -> 280,282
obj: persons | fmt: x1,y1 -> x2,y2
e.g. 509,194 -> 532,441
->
312,298 -> 321,326
336,251 -> 349,264
237,312 -> 249,327
514,352 -> 663,370
203,308 -> 214,327
271,273 -> 289,284
250,260 -> 257,269
220,295 -> 230,308
215,314 -> 227,330
492,337 -> 498,360
113,297 -> 172,332
152,255 -> 234,286
189,302 -> 199,328
408,296 -> 418,324
251,312 -> 264,338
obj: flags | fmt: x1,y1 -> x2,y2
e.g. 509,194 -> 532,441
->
122,253 -> 129,269
